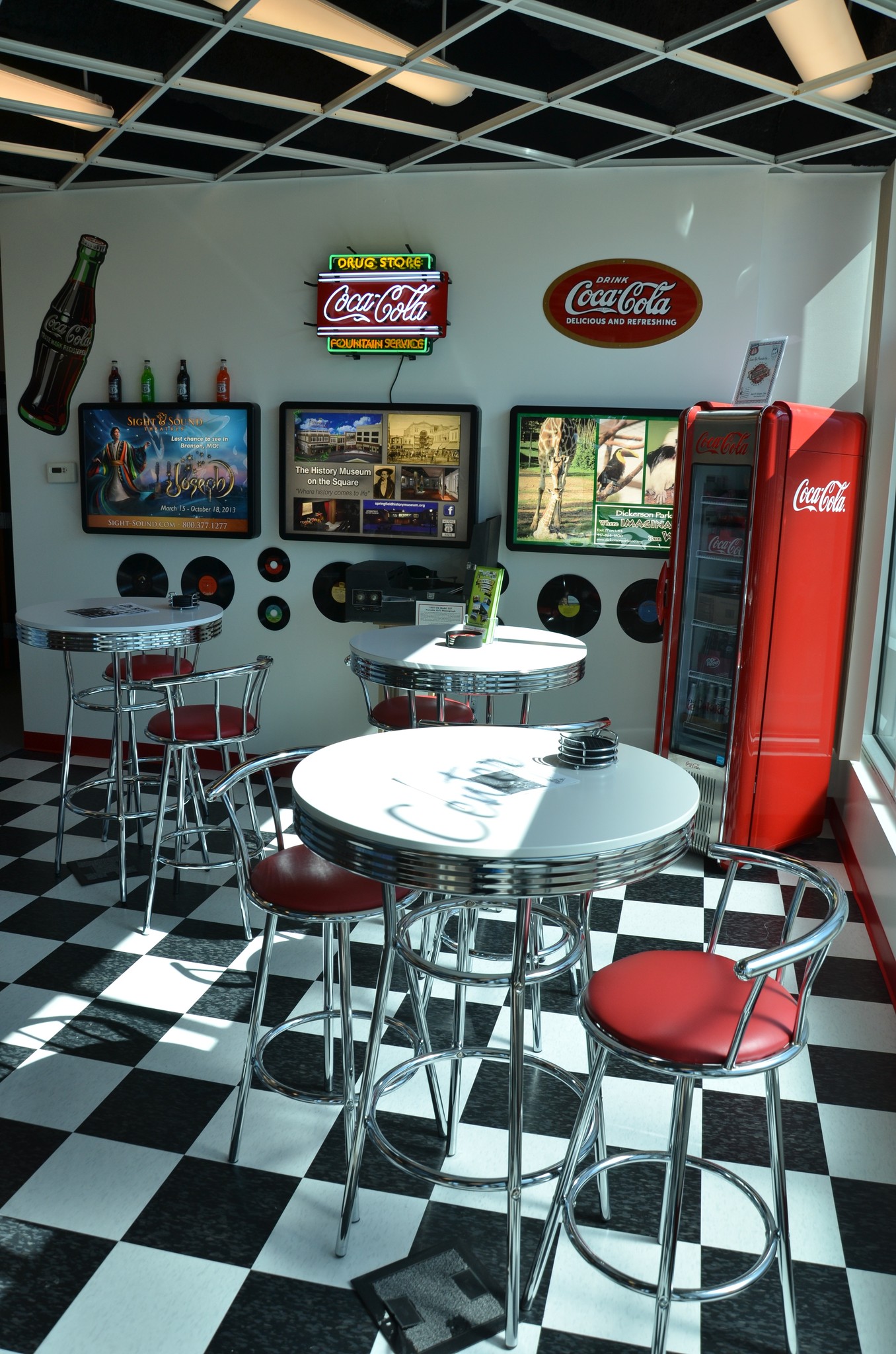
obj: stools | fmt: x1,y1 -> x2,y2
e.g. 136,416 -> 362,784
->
60,641 -> 850,1354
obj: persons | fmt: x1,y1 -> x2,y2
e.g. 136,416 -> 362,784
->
406,484 -> 408,488
420,481 -> 423,492
374,468 -> 395,499
87,427 -> 155,517
413,519 -> 417,527
408,483 -> 410,488
444,484 -> 447,494
433,483 -> 435,489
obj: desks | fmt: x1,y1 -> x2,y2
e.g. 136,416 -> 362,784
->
16,595 -> 224,906
350,624 -> 588,727
291,724 -> 702,1346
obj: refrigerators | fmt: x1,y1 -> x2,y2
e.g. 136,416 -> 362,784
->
654,401 -> 871,872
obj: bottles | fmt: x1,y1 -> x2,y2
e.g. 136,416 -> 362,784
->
177,359 -> 190,402
141,360 -> 154,402
685,680 -> 732,724
216,359 -> 230,402
108,361 -> 122,403
17,234 -> 109,436
698,630 -> 736,676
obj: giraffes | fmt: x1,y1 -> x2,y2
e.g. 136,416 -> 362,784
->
529,417 -> 578,530
532,489 -> 586,538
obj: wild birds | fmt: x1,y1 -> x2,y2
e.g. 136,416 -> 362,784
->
598,447 -> 639,492
